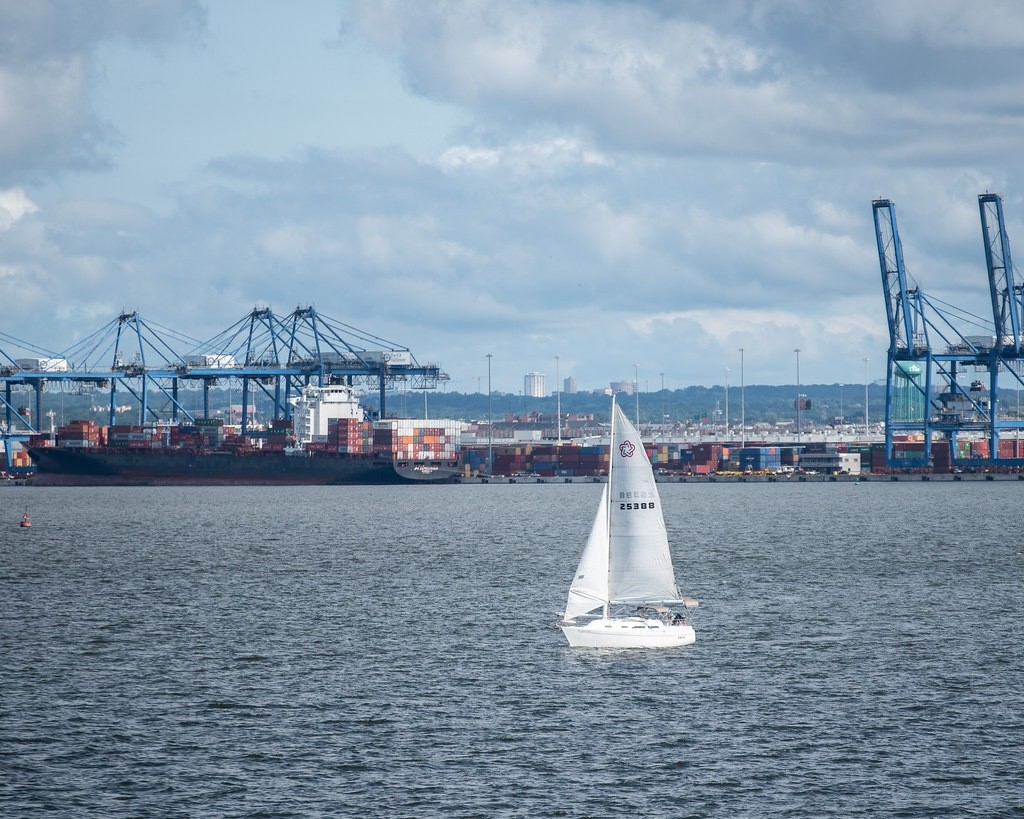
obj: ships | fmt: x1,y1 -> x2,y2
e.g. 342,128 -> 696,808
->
26,420 -> 462,484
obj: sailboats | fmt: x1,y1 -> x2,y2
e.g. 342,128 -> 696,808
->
554,393 -> 700,652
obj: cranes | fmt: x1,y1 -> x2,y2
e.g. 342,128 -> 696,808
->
0,304 -> 452,424
871,190 -> 1024,471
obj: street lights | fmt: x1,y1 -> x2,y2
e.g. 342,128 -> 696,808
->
862,356 -> 871,437
632,362 -> 640,436
660,372 -> 664,428
485,353 -> 492,473
724,367 -> 731,440
554,354 -> 561,445
838,384 -> 844,428
739,346 -> 745,448
793,347 -> 802,443
